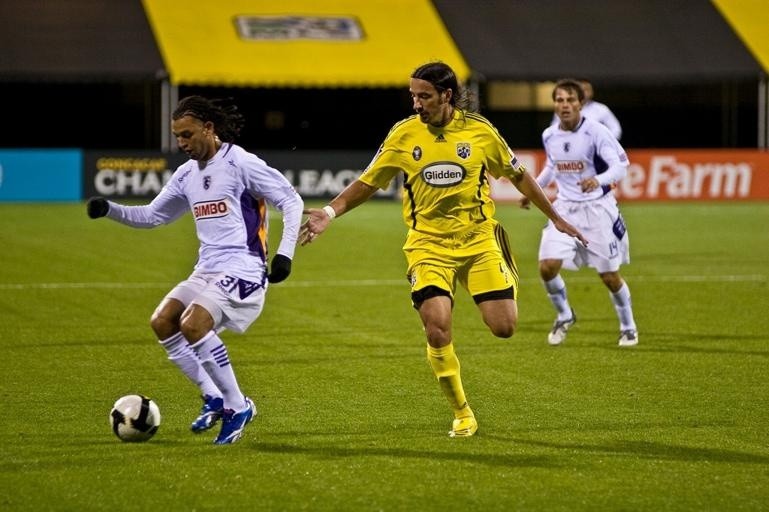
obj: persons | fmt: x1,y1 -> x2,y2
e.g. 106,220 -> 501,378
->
517,80 -> 639,347
292,57 -> 592,438
83,96 -> 305,445
549,76 -> 624,197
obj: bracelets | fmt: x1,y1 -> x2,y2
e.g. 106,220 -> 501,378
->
322,204 -> 336,220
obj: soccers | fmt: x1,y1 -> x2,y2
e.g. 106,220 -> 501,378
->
110,394 -> 160,443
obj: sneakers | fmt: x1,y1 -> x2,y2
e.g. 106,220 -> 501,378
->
547,308 -> 576,346
190,394 -> 224,433
212,397 -> 257,445
452,411 -> 479,438
618,328 -> 639,346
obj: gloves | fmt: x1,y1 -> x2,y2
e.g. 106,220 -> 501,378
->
267,254 -> 291,283
87,197 -> 109,219
